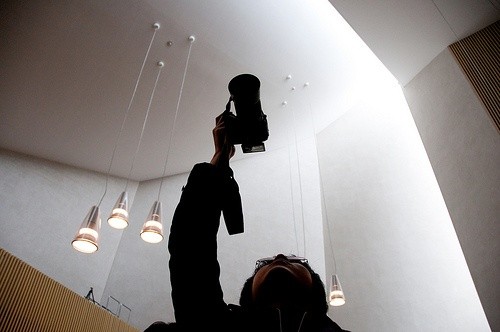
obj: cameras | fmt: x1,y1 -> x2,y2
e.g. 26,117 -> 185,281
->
221,73 -> 269,153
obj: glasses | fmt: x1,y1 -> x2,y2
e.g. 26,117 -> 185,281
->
253,255 -> 316,278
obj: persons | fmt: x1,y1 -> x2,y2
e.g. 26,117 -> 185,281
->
168,116 -> 351,332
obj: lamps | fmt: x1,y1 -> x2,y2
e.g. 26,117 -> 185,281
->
319,168 -> 345,306
107,61 -> 165,229
71,23 -> 160,253
140,36 -> 195,244
